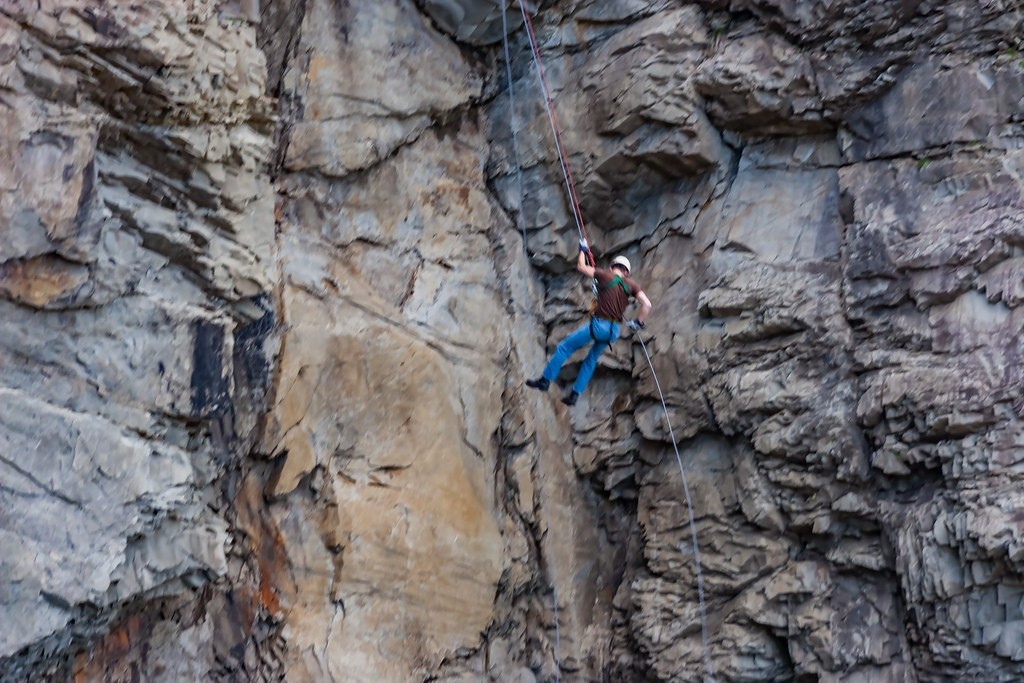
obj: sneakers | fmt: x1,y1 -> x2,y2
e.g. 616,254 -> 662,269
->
525,376 -> 549,392
561,394 -> 577,405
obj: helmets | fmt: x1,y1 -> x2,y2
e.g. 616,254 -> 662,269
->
612,255 -> 631,273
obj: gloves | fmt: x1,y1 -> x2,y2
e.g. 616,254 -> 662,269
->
579,239 -> 587,252
627,320 -> 643,330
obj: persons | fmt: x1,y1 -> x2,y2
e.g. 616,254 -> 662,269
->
523,238 -> 651,404
